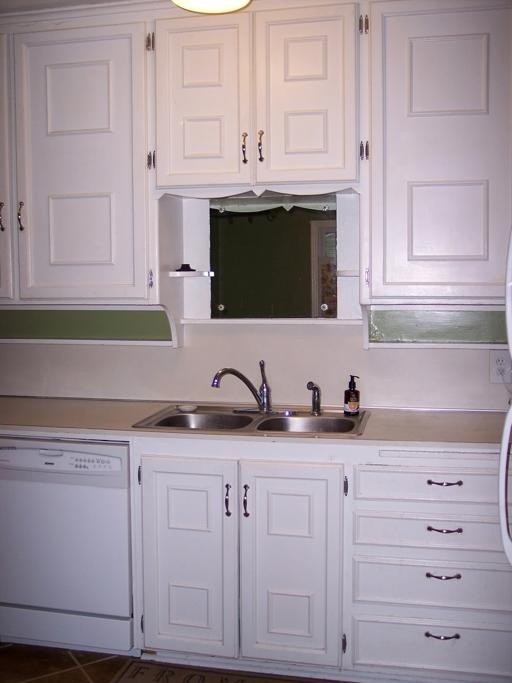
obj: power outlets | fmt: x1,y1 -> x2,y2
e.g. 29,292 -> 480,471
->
488,350 -> 512,385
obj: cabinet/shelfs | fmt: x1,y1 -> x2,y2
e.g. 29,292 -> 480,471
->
347,418 -> 512,682
143,0 -> 355,191
0,0 -> 183,346
356,0 -> 508,346
135,422 -> 348,682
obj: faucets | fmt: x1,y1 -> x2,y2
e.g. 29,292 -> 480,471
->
210,359 -> 272,411
306,381 -> 321,415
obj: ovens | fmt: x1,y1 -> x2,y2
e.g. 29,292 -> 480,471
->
0,435 -> 135,656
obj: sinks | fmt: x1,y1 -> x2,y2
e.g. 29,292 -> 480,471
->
155,414 -> 253,430
257,416 -> 355,433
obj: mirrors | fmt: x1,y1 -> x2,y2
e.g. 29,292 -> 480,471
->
202,187 -> 339,320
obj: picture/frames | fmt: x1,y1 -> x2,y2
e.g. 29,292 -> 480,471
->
310,219 -> 337,319
